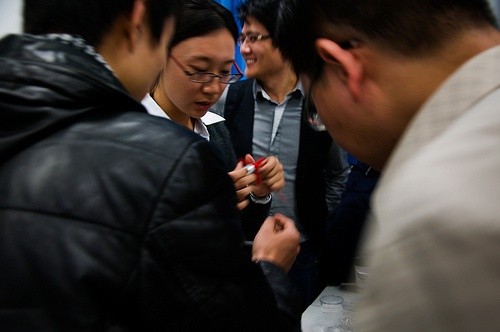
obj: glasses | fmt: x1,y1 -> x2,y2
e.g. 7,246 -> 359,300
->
302,36 -> 362,132
167,53 -> 244,85
236,32 -> 274,46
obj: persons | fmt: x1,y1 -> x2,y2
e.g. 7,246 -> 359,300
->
0,0 -> 500,332
136,0 -> 285,257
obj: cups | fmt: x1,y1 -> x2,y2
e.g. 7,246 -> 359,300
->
355,267 -> 370,292
319,295 -> 345,324
